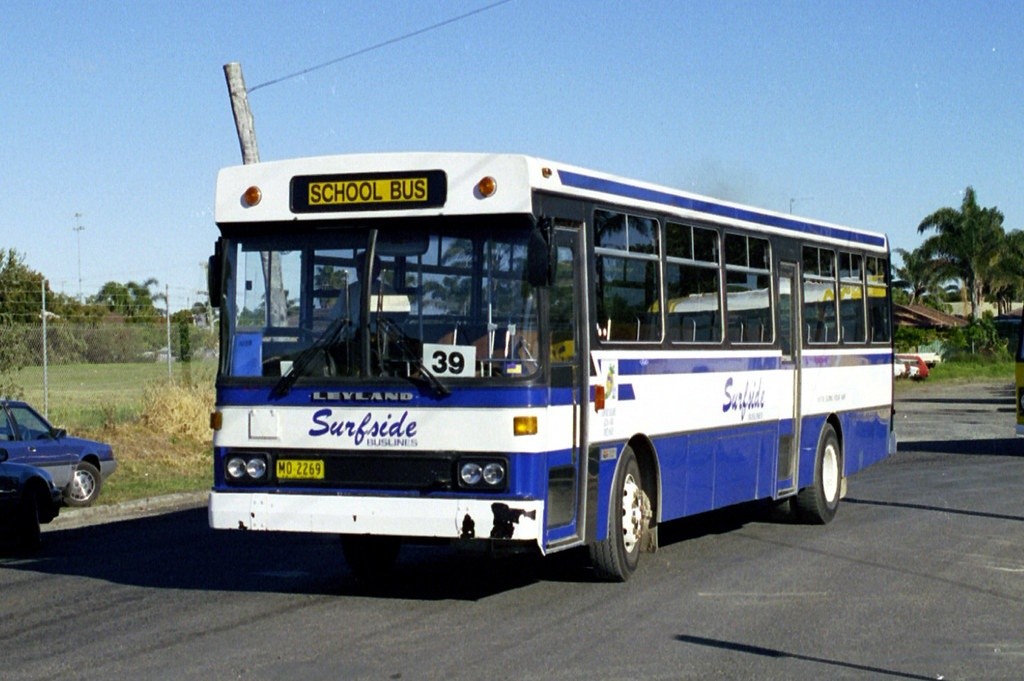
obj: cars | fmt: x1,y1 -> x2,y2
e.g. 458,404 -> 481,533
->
0,448 -> 60,551
0,402 -> 117,507
894,353 -> 930,380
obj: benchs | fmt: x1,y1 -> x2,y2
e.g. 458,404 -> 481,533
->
398,312 -> 847,377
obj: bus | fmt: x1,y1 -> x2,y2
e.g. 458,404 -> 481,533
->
205,149 -> 899,584
